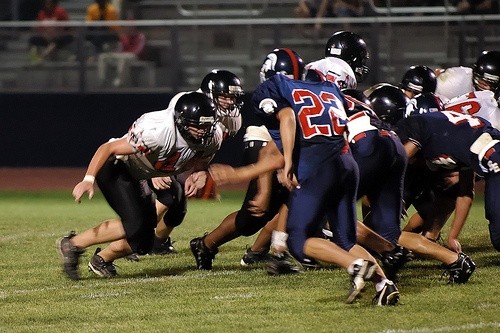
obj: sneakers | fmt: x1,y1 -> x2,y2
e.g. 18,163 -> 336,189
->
55,230 -> 86,282
267,252 -> 307,277
371,279 -> 400,306
445,252 -> 476,287
344,259 -> 378,304
241,247 -> 270,269
87,248 -> 121,279
380,242 -> 413,281
123,252 -> 141,262
189,236 -> 216,271
150,233 -> 177,257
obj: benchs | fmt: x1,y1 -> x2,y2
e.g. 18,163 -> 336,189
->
17,40 -> 170,84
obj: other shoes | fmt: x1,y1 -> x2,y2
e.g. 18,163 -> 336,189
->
288,249 -> 322,268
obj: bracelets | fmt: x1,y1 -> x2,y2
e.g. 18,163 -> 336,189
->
83,175 -> 95,183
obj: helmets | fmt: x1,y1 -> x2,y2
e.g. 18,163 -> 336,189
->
173,92 -> 217,144
259,47 -> 307,81
325,30 -> 370,80
201,70 -> 246,109
363,83 -> 407,125
338,88 -> 375,118
399,66 -> 437,96
472,50 -> 500,94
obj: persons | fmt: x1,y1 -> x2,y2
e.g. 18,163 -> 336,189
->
84,0 -> 121,54
61,31 -> 500,307
297,0 -> 378,39
94,12 -> 146,88
449,0 -> 493,14
23,0 -> 74,64
